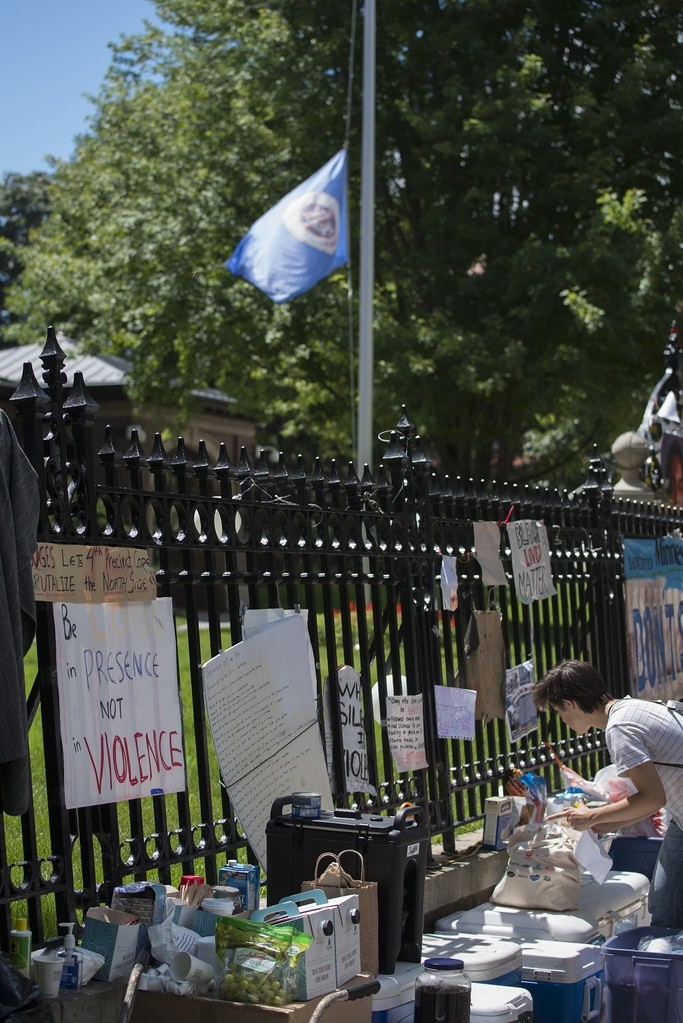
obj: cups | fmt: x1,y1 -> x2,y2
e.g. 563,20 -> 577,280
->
32,955 -> 65,999
200,897 -> 236,919
168,952 -> 214,984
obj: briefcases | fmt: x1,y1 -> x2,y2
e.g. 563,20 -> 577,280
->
435,865 -> 653,946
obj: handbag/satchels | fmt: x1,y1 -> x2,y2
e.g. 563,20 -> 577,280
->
300,849 -> 378,979
488,837 -> 581,911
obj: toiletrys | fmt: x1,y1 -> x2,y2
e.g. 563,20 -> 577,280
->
56,922 -> 82,998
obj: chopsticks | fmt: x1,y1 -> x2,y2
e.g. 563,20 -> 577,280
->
176,880 -> 212,907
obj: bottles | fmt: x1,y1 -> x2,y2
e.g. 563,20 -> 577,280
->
8,917 -> 32,984
413,957 -> 472,1023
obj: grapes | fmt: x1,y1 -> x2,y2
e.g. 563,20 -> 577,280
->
219,961 -> 296,1006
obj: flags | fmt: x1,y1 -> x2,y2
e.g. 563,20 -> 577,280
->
225,149 -> 349,304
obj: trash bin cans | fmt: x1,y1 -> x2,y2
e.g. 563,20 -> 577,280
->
602,926 -> 683,1023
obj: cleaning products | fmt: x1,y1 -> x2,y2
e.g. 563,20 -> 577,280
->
9,918 -> 32,981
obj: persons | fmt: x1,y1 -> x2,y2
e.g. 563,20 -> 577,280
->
533,660 -> 683,930
